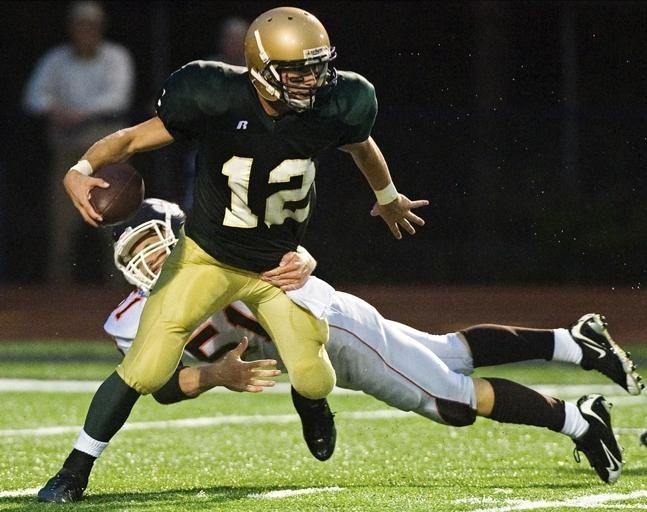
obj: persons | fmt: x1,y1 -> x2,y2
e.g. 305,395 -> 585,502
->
104,197 -> 643,484
37,5 -> 430,502
179,19 -> 250,206
25,5 -> 133,293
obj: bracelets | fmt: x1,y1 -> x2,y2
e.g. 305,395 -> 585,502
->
374,181 -> 399,205
67,159 -> 93,177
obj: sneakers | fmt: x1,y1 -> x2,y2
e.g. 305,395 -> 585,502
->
291,385 -> 337,462
568,312 -> 643,395
39,464 -> 85,504
572,393 -> 626,485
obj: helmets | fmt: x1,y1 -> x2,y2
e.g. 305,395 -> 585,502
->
244,7 -> 337,115
112,197 -> 188,294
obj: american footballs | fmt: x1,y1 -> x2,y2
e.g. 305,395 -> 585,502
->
89,163 -> 144,227
